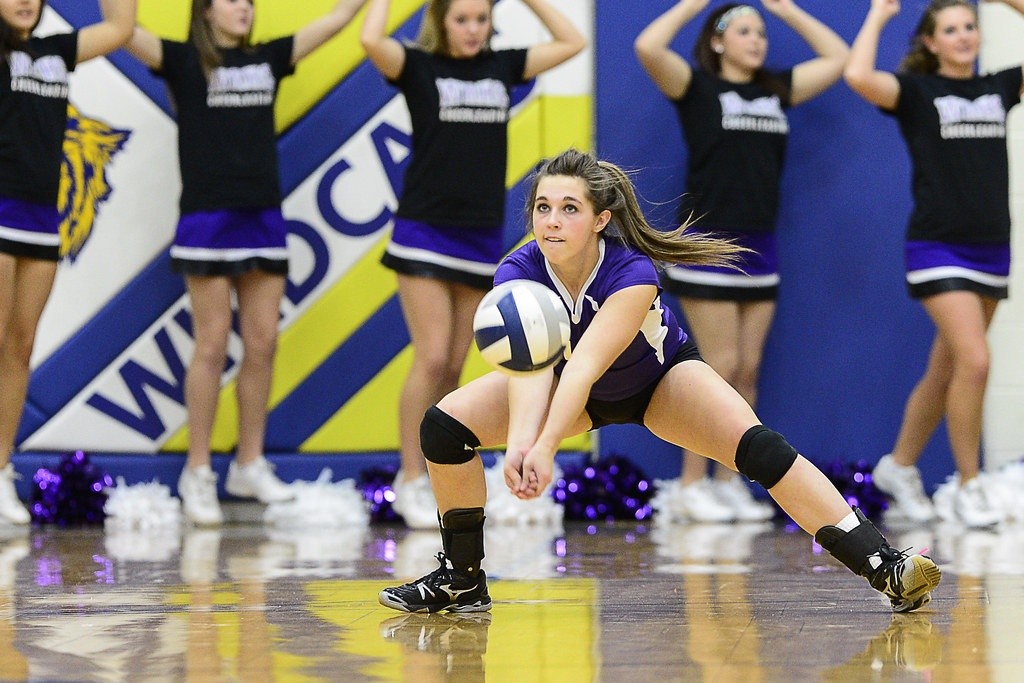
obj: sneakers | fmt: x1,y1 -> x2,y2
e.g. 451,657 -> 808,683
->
952,479 -> 1000,530
881,612 -> 942,671
666,478 -> 775,523
389,473 -> 442,529
177,466 -> 225,527
0,464 -> 32,525
224,459 -> 296,502
378,551 -> 492,612
379,612 -> 492,655
871,455 -> 936,524
869,548 -> 940,613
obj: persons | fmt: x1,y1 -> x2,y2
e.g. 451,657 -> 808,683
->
375,146 -> 943,615
356,0 -> 590,532
840,1 -> 1024,533
97,2 -> 365,526
633,1 -> 851,527
0,1 -> 136,525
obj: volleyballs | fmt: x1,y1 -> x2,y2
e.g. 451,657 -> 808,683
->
471,277 -> 572,378
